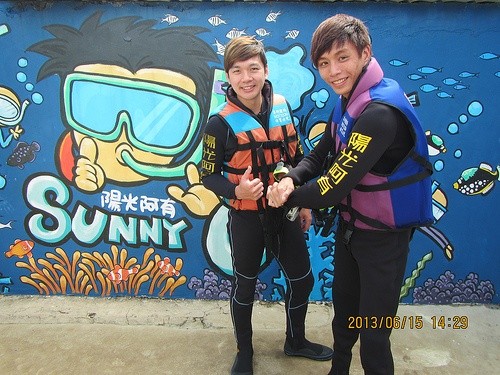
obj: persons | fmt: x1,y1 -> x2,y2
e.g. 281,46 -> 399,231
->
201,36 -> 334,375
268,12 -> 434,375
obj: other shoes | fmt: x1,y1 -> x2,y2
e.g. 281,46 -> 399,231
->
284,336 -> 334,361
230,348 -> 253,375
